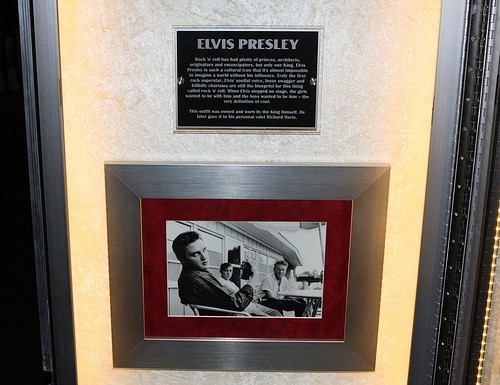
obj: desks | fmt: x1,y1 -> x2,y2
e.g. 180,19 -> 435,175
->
278,289 -> 322,317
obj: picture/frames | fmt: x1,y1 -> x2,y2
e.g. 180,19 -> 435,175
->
105,161 -> 391,372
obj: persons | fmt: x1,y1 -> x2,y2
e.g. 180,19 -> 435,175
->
257,259 -> 309,319
218,260 -> 242,296
170,229 -> 282,320
